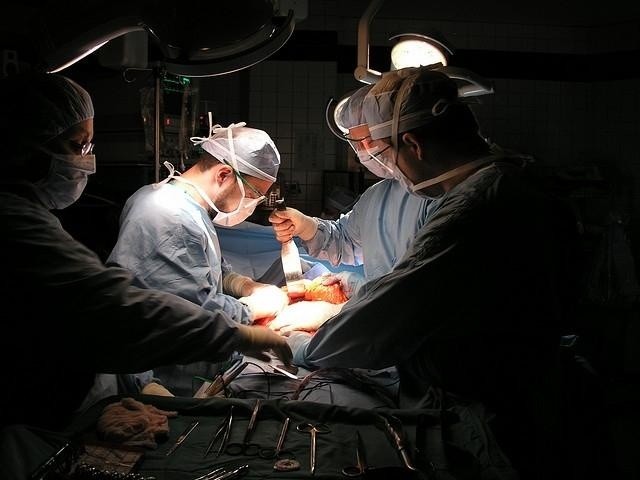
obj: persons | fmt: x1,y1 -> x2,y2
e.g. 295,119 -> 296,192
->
0,74 -> 289,429
281,67 -> 640,479
104,122 -> 289,397
268,83 -> 437,301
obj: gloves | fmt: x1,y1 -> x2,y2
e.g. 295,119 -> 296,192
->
222,271 -> 289,320
269,301 -> 340,335
268,207 -> 319,241
240,324 -> 293,365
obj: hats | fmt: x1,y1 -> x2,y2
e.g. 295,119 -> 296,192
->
331,68 -> 458,140
202,126 -> 281,183
19,75 -> 94,145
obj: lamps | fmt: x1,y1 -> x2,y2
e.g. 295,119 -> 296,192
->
354,0 -> 496,98
34,0 -> 296,78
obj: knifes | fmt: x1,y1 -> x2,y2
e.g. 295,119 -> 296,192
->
165,420 -> 199,457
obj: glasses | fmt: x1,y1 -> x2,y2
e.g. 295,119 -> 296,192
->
347,137 -> 390,160
223,159 -> 269,204
80,142 -> 95,157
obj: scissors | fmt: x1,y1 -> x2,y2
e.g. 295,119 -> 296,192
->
258,418 -> 296,461
341,430 -> 377,479
296,423 -> 331,476
226,399 -> 261,456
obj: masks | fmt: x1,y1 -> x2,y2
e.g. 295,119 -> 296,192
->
357,149 -> 448,201
211,167 -> 258,228
19,140 -> 96,210
